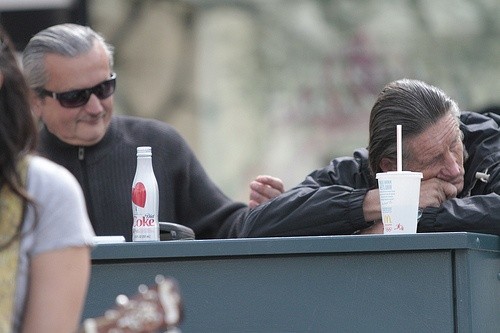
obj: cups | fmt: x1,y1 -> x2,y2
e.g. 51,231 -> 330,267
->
376,171 -> 423,234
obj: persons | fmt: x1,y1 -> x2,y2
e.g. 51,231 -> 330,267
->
22,22 -> 286,242
0,26 -> 97,333
226,79 -> 500,237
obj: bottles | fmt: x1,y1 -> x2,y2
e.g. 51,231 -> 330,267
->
132,146 -> 160,242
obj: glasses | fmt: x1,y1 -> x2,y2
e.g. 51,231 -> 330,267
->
40,72 -> 116,108
